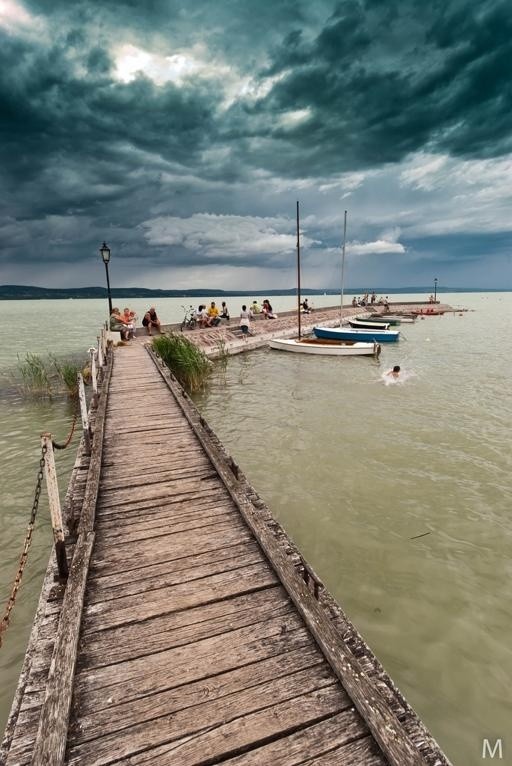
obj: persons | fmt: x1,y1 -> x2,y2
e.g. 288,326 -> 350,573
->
429,295 -> 434,304
303,298 -> 312,311
190,298 -> 273,342
110,307 -> 137,340
142,307 -> 165,336
387,366 -> 399,379
352,291 -> 391,314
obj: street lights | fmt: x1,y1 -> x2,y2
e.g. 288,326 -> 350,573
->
99,241 -> 113,316
434,277 -> 438,302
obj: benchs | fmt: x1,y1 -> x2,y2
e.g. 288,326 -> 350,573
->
108,331 -> 121,345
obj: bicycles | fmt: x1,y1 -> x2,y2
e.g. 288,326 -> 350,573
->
180,305 -> 196,332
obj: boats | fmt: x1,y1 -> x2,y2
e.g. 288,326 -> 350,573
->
371,312 -> 417,324
355,317 -> 400,325
312,326 -> 400,342
267,338 -> 382,356
411,311 -> 444,315
345,319 -> 390,330
